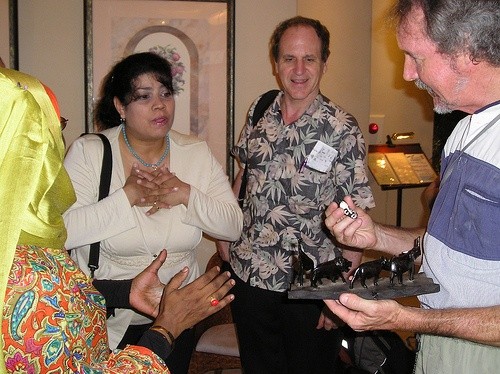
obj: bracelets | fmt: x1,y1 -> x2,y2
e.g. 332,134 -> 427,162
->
149,326 -> 174,348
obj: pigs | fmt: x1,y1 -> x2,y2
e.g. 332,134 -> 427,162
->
309,255 -> 353,288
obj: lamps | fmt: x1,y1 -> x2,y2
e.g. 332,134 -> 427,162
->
386,131 -> 415,148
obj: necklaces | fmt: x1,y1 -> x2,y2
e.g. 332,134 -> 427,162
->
122,123 -> 170,168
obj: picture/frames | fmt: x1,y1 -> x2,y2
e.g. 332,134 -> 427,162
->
0,0 -> 19,71
83,0 -> 236,190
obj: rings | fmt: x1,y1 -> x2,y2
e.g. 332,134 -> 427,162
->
209,295 -> 218,308
154,203 -> 159,210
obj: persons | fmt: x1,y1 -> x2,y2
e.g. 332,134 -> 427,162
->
61,52 -> 243,374
323,1 -> 500,374
0,66 -> 236,374
216,15 -> 377,374
